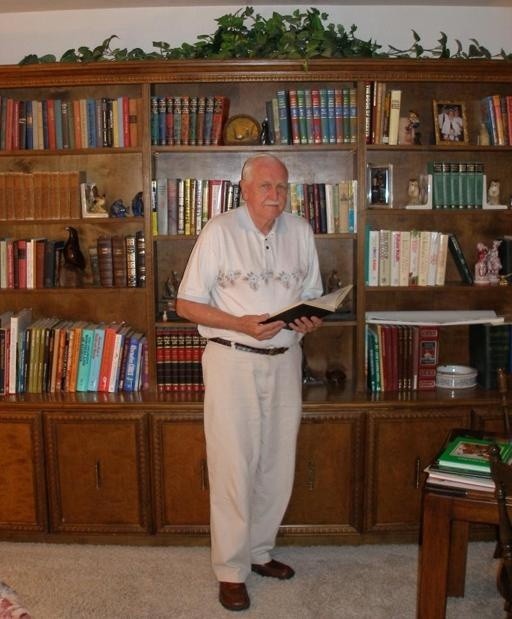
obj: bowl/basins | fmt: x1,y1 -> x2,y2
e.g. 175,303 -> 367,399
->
435,364 -> 480,389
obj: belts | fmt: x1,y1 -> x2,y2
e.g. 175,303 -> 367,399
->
210,336 -> 288,356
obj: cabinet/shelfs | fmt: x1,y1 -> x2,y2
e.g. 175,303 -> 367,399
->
0,57 -> 512,547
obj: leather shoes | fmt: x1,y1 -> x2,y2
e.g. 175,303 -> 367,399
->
251,560 -> 295,579
218,581 -> 250,610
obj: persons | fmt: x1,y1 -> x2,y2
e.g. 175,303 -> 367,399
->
474,239 -> 503,281
175,153 -> 321,612
439,106 -> 465,142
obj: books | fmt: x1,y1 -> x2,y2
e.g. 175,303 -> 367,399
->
257,282 -> 354,331
282,180 -> 357,234
366,81 -> 401,146
150,179 -> 245,237
0,230 -> 146,289
0,308 -> 148,396
426,159 -> 485,210
468,323 -> 511,391
155,327 -> 208,392
480,94 -> 510,146
0,171 -> 87,220
364,222 -> 473,286
363,323 -> 438,392
0,97 -> 140,150
150,97 -> 231,145
427,432 -> 512,497
266,89 -> 357,145
